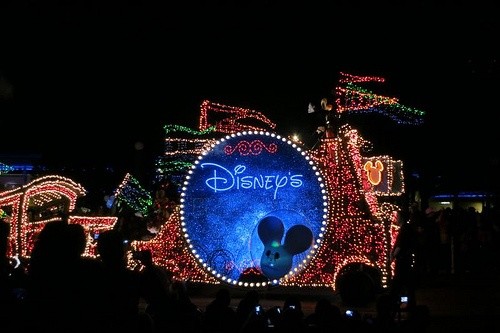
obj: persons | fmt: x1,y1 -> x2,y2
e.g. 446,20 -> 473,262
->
1,196 -> 500,333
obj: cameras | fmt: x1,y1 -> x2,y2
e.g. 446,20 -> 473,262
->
133,251 -> 144,259
94,233 -> 100,241
